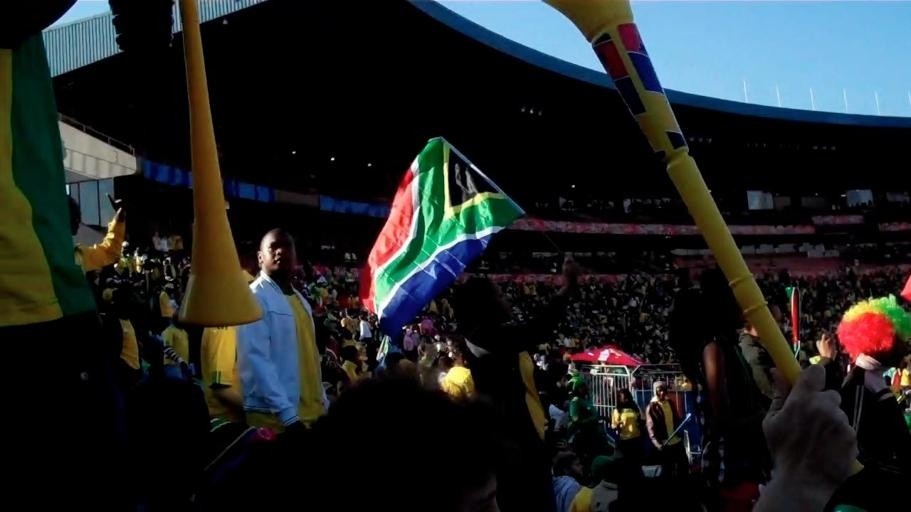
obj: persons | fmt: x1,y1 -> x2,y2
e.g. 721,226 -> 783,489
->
576,271 -> 680,344
835,296 -> 911,511
359,317 -> 373,354
66,193 -> 127,277
295,262 -> 360,338
440,358 -> 477,404
115,234 -> 192,293
341,346 -> 364,388
741,305 -> 837,405
375,354 -> 403,385
751,365 -> 856,511
648,381 -> 684,464
405,329 -> 416,359
100,290 -> 142,382
235,228 -> 328,443
159,284 -> 178,327
794,268 -> 910,307
417,299 -> 467,363
613,388 -> 642,446
0,0 -> 98,427
200,269 -> 258,427
757,272 -> 793,306
164,310 -> 193,382
396,359 -> 416,381
569,377 -> 599,455
551,454 -> 595,511
431,454 -> 501,511
455,257 -> 578,446
791,306 -> 837,363
323,340 -> 340,367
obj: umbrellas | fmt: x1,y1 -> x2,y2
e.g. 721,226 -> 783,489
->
566,345 -> 643,367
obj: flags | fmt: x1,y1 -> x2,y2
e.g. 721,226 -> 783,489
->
361,136 -> 528,341
